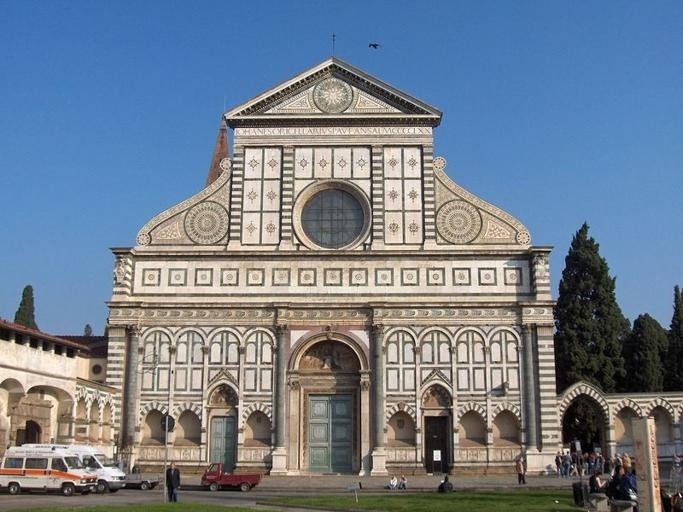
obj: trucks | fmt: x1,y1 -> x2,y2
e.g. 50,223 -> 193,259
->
200,462 -> 262,493
110,458 -> 160,491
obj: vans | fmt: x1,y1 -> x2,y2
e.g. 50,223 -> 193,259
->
0,444 -> 98,496
21,441 -> 127,495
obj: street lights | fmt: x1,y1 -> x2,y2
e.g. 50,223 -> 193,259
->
111,436 -> 119,458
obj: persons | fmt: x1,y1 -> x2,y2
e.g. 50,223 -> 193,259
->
670,453 -> 681,473
385,474 -> 397,489
398,476 -> 408,490
588,464 -> 682,512
438,475 -> 452,493
165,460 -> 180,501
514,456 -> 525,485
553,451 -> 631,479
